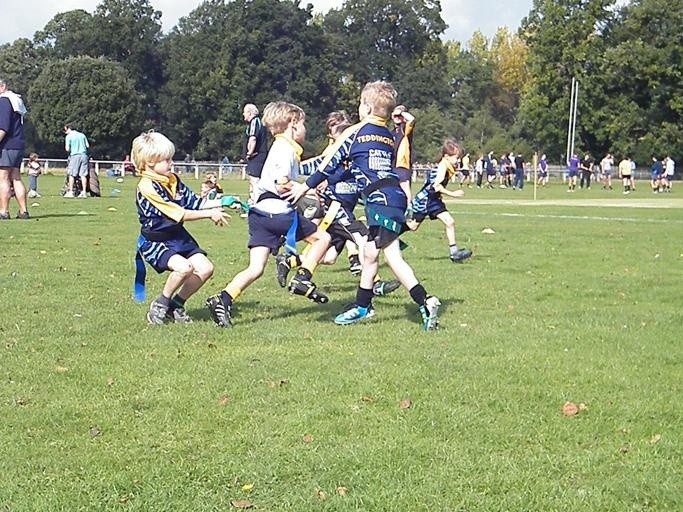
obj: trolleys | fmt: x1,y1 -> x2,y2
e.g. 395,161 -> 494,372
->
59,178 -> 80,197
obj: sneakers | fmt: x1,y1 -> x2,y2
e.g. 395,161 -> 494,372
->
458,180 -> 674,195
204,294 -> 235,329
165,304 -> 194,323
287,275 -> 329,304
0,211 -> 10,219
418,293 -> 441,333
450,248 -> 473,262
275,253 -> 292,288
27,189 -> 41,198
147,297 -> 169,325
347,262 -> 363,276
334,302 -> 377,325
62,191 -> 75,198
76,192 -> 88,198
15,209 -> 29,219
371,279 -> 402,295
